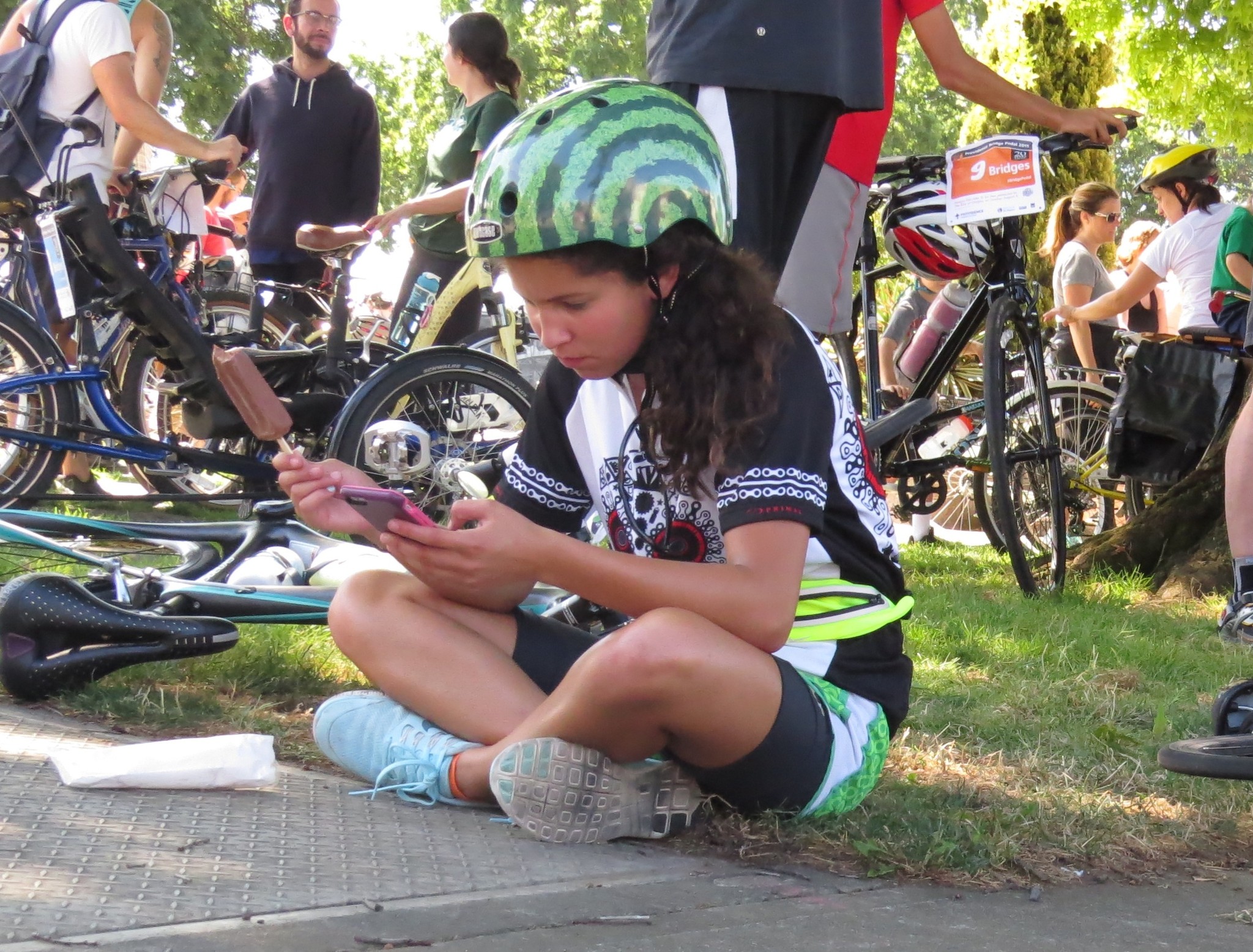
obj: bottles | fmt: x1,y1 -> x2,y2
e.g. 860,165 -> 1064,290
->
918,415 -> 974,459
538,594 -> 634,635
391,271 -> 442,347
899,281 -> 973,382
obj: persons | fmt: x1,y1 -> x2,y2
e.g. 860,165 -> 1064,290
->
271,76 -> 912,849
0,0 -> 1253,642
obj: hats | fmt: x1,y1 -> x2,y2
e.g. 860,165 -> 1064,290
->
226,196 -> 254,215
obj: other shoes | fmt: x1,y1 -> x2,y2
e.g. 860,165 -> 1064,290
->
1064,523 -> 1082,548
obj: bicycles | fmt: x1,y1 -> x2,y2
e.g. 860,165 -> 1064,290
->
0,115 -> 567,704
824,114 -> 1193,600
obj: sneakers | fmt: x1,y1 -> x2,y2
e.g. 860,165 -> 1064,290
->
488,738 -> 706,848
56,470 -> 122,509
1216,591 -> 1253,643
311,689 -> 496,807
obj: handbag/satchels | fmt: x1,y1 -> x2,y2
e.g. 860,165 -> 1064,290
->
1107,340 -> 1245,493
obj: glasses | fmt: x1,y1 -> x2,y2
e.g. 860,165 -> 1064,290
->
1080,206 -> 1123,223
292,10 -> 343,28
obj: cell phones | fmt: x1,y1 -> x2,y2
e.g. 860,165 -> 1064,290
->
340,485 -> 446,540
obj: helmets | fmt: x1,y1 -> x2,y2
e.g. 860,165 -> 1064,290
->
465,76 -> 733,261
347,315 -> 392,344
880,178 -> 991,283
1132,142 -> 1219,197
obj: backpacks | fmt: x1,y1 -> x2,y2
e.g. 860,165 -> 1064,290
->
0,0 -> 102,192
